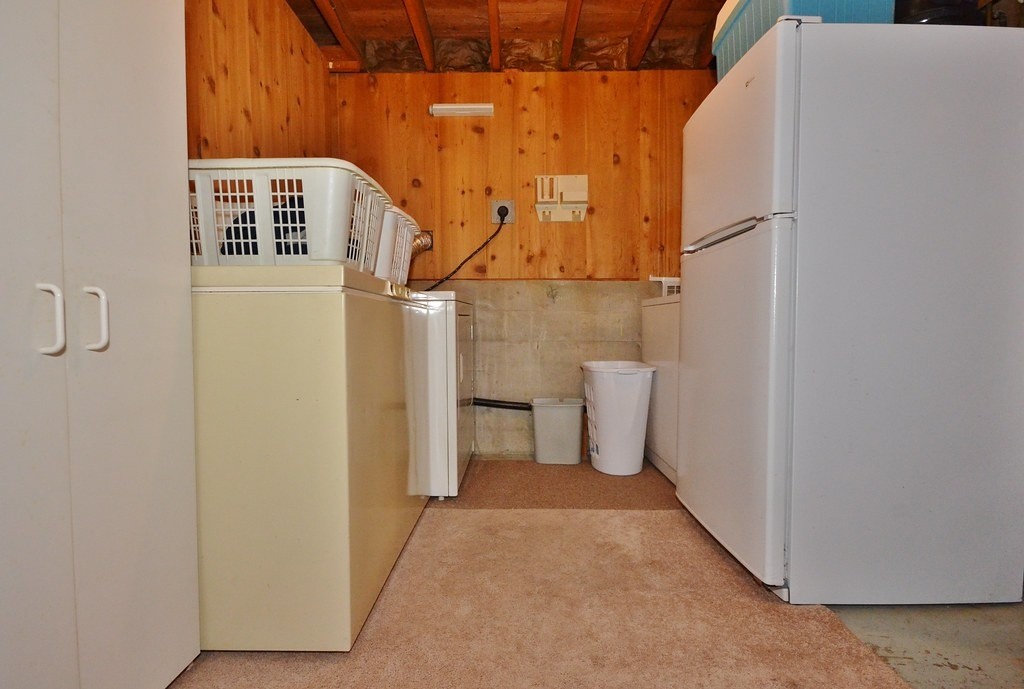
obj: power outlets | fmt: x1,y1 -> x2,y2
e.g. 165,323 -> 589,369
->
492,200 -> 514,224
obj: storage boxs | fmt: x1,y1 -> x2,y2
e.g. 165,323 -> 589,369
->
712,0 -> 895,84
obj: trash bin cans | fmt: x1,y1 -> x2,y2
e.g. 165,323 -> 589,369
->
529,397 -> 586,464
581,360 -> 656,476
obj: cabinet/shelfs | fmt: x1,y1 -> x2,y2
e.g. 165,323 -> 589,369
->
0,0 -> 206,689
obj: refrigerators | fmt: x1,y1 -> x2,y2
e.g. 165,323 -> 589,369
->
670,21 -> 1024,604
190,263 -> 475,651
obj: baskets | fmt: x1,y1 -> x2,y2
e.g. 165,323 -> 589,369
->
188,157 -> 393,276
373,206 -> 421,287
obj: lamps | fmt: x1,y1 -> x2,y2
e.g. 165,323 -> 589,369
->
429,103 -> 495,117
